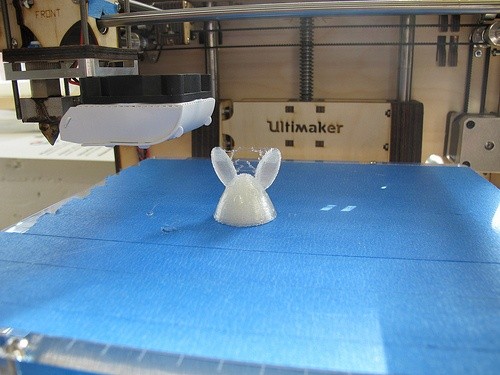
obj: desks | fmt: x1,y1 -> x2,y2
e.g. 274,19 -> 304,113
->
1,156 -> 500,375
